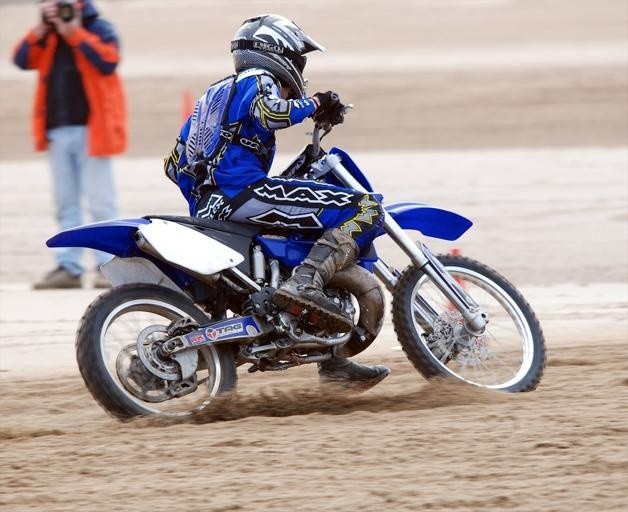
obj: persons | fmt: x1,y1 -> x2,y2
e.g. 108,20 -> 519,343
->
165,15 -> 390,396
12,1 -> 128,290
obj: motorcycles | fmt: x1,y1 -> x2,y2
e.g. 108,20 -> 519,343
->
46,90 -> 548,423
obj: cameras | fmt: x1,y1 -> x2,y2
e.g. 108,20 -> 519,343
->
58,2 -> 73,21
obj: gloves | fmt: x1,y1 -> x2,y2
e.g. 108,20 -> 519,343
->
308,89 -> 345,127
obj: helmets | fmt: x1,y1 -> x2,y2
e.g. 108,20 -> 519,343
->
230,14 -> 323,99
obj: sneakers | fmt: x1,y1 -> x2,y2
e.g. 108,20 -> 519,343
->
316,358 -> 391,397
272,227 -> 360,333
34,263 -> 112,289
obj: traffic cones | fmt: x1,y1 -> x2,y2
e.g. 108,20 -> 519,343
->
182,89 -> 197,125
447,248 -> 484,353
